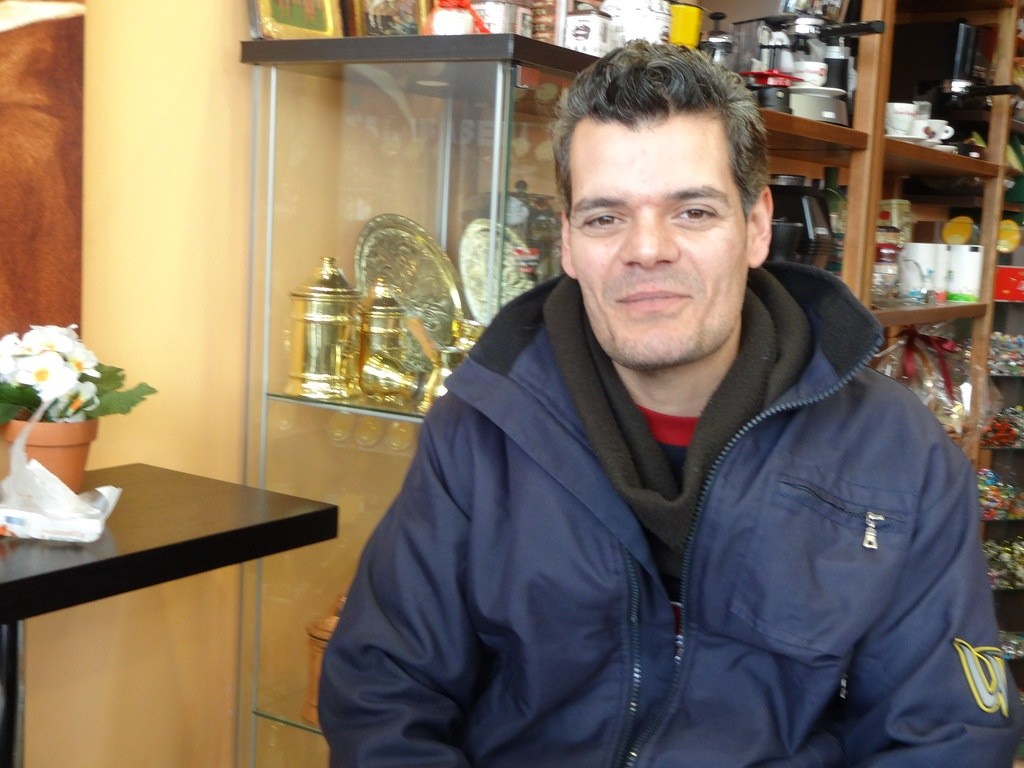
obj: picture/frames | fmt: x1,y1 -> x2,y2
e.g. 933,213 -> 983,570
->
256,1 -> 343,40
345,1 -> 432,39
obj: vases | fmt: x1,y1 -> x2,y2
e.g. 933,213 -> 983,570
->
4,416 -> 98,499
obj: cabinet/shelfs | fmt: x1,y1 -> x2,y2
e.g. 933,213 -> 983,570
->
235,1 -> 1024,766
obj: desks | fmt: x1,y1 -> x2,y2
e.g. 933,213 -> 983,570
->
0,461 -> 340,628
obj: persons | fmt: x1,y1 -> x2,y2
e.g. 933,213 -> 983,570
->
317,39 -> 1023,768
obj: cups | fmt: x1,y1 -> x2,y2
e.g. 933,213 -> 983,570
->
885,103 -> 916,134
917,120 -> 955,149
791,61 -> 829,87
869,250 -> 902,309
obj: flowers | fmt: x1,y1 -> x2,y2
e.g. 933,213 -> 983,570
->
0,324 -> 158,428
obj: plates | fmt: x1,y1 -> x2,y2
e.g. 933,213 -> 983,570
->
790,86 -> 847,99
883,134 -> 924,146
